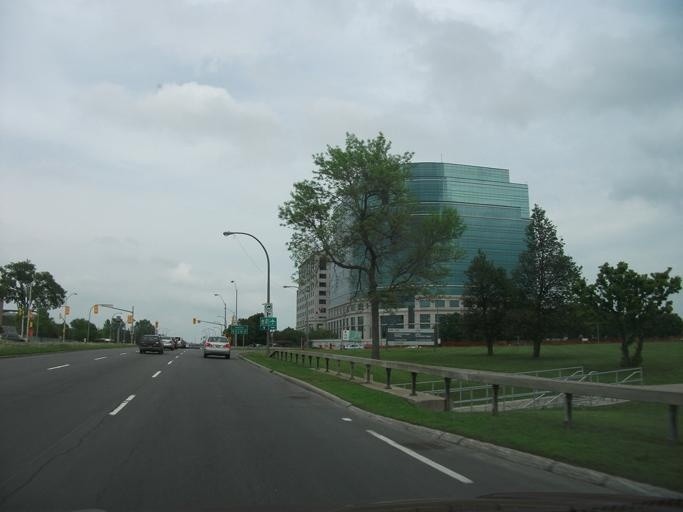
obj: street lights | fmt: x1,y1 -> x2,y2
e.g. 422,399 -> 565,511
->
223,232 -> 270,345
62,292 -> 77,341
283,285 -> 309,349
215,293 -> 227,329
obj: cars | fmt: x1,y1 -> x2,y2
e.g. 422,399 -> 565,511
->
0,332 -> 24,342
139,335 -> 164,353
204,336 -> 231,359
161,336 -> 186,350
344,343 -> 382,348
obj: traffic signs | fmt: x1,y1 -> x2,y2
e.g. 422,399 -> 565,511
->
260,317 -> 277,329
230,325 -> 248,335
